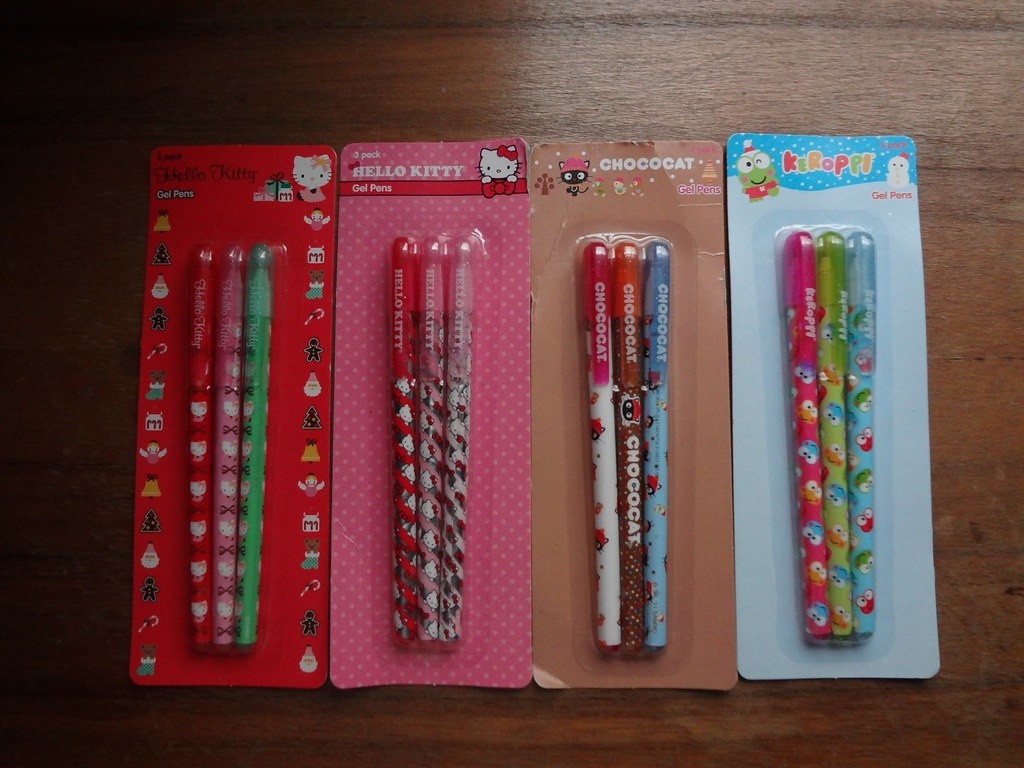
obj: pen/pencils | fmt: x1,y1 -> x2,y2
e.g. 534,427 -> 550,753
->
781,227 -> 880,641
576,238 -> 673,655
183,244 -> 274,650
387,236 -> 477,649
